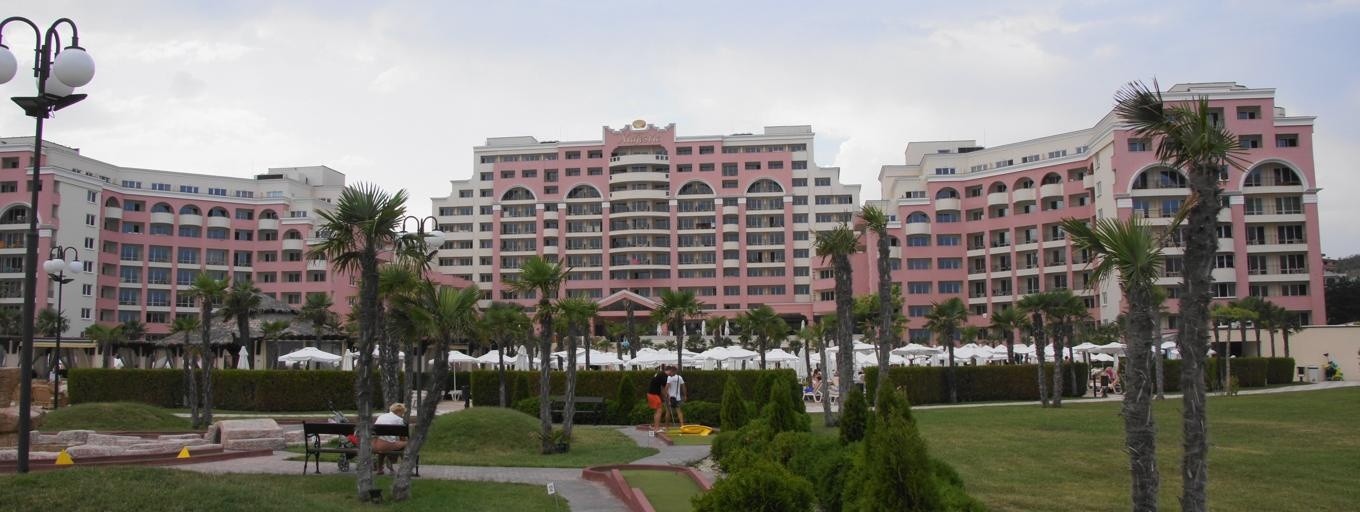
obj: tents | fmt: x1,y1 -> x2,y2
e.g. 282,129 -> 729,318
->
277,340 -> 1182,391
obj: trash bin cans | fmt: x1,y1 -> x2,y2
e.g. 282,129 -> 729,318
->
1307,366 -> 1319,383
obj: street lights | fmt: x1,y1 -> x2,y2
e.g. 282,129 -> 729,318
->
44,244 -> 86,409
1,12 -> 93,473
394,213 -> 447,427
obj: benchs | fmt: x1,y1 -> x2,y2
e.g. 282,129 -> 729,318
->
550,396 -> 607,425
301,420 -> 419,478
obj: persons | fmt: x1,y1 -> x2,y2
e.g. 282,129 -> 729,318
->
369,402 -> 409,475
665,366 -> 688,429
646,366 -> 672,433
1106,367 -> 1120,394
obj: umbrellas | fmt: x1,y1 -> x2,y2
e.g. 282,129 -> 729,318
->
237,345 -> 250,369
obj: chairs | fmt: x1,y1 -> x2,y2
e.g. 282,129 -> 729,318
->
802,377 -> 866,405
16,380 -> 69,408
